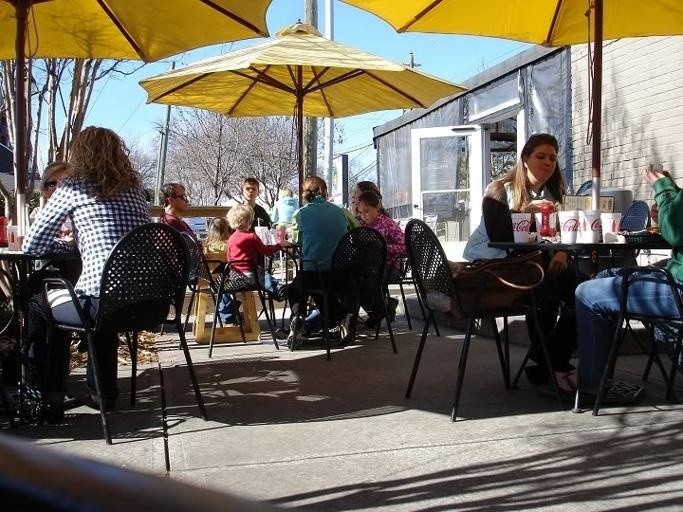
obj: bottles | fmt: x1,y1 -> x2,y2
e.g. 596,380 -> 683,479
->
270,224 -> 286,245
0,208 -> 8,247
540,203 -> 557,242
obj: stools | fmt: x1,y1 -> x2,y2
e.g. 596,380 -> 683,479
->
593,267 -> 683,417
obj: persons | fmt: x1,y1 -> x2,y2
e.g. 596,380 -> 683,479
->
20,125 -> 170,423
351,190 -> 407,327
556,163 -> 683,399
205,178 -> 288,327
161,182 -> 204,284
462,133 -> 591,385
352,180 -> 391,218
286,176 -> 368,351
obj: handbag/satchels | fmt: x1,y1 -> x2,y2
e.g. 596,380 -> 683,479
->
454,250 -> 544,313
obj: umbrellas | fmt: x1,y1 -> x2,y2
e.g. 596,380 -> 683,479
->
138,17 -> 468,208
338,0 -> 683,210
1,0 -> 272,248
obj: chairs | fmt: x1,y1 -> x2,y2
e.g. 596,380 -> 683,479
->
36,223 -> 208,476
179,232 -> 281,358
405,219 -> 565,422
291,227 -> 400,362
617,199 -> 651,257
372,231 -> 441,354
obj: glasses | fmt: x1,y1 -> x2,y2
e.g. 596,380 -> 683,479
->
175,194 -> 187,201
46,181 -> 57,186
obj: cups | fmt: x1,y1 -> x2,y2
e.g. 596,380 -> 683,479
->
511,211 -> 622,244
254,226 -> 292,246
18,236 -> 25,251
7,226 -> 19,251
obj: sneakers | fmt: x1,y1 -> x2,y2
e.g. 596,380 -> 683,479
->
388,298 -> 397,322
339,314 -> 355,344
287,316 -> 304,350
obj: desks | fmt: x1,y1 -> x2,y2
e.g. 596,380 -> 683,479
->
251,244 -> 302,335
0,248 -> 81,428
488,240 -> 672,407
